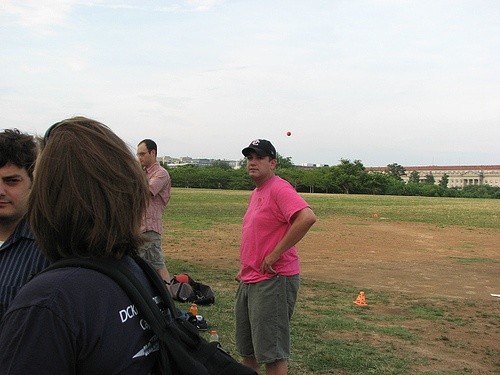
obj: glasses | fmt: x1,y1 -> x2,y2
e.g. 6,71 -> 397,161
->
136,151 -> 149,156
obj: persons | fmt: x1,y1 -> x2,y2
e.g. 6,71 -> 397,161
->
0,128 -> 49,325
137,139 -> 170,297
0,116 -> 182,375
235,139 -> 316,375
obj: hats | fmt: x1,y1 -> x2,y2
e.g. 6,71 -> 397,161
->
242,139 -> 276,157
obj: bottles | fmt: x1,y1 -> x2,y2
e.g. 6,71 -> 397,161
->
210,331 -> 218,347
190,304 -> 197,316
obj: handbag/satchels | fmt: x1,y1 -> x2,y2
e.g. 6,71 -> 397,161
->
164,273 -> 215,330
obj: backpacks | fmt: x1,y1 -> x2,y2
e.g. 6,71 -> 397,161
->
34,249 -> 260,375
